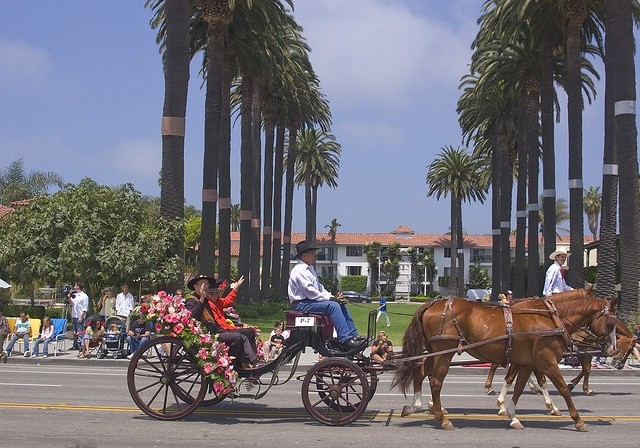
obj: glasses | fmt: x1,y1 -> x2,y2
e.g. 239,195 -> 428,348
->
197,282 -> 211,287
279,324 -> 283,326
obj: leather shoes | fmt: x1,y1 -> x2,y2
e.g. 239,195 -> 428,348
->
69,347 -> 79,350
354,336 -> 366,341
343,339 -> 365,352
253,361 -> 270,367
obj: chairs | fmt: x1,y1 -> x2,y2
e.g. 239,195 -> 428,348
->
126,321 -> 154,357
16,318 -> 41,357
48,319 -> 68,357
3,316 -> 17,354
77,336 -> 105,359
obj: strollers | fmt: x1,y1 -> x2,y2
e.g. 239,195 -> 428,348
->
96,315 -> 128,359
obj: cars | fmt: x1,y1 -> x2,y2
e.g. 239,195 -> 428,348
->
340,291 -> 373,303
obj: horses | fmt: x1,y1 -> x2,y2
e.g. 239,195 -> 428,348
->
484,318 -> 640,395
401,284 -> 594,418
389,295 -> 617,432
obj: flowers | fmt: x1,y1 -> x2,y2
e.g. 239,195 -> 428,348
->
130,291 -> 239,398
223,306 -> 267,383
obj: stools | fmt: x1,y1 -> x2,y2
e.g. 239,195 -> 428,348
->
287,311 -> 333,339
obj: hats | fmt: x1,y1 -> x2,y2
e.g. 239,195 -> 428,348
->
506,290 -> 514,295
292,241 -> 320,260
208,278 -> 223,293
187,274 -> 216,291
549,247 -> 571,260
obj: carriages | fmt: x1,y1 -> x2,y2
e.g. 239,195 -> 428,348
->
128,284 -> 619,432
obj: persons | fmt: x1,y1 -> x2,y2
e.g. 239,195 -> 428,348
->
206,275 -> 263,369
106,322 -> 121,340
78,317 -> 105,359
104,291 -> 116,321
1,311 -> 32,357
32,317 -> 54,358
176,289 -> 186,304
506,290 -> 515,303
543,247 -> 575,296
126,315 -> 150,359
370,331 -> 393,366
67,281 -> 89,350
265,320 -> 290,363
0,307 -> 11,358
498,293 -> 508,304
375,293 -> 391,327
288,241 -> 369,352
269,326 -> 284,360
116,283 -> 134,322
97,287 -> 110,321
184,273 -> 246,370
633,332 -> 640,362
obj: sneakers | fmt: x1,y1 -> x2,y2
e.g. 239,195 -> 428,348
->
2,351 -> 11,356
85,352 -> 89,356
80,353 -> 86,357
32,355 -> 36,357
24,351 -> 31,357
43,355 -> 47,358
127,353 -> 134,359
